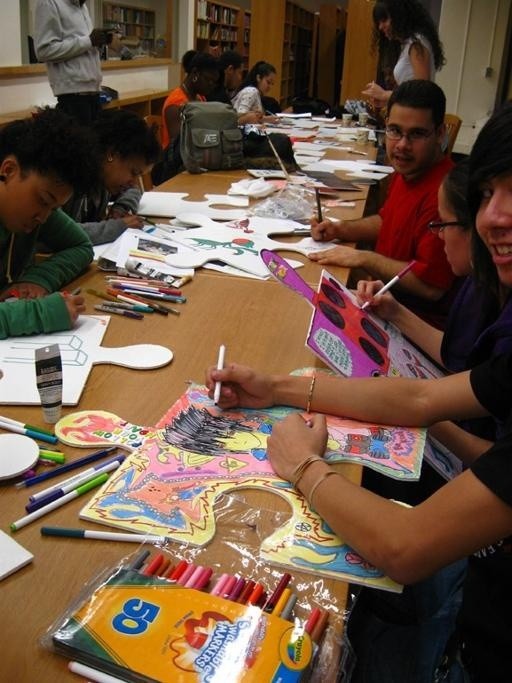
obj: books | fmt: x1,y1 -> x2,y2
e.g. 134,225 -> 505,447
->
193,0 -> 252,45
104,3 -> 157,40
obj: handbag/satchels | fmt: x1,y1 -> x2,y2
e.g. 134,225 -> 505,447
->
292,95 -> 328,116
178,101 -> 243,174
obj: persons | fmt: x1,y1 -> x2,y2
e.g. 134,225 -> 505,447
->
349,156 -> 504,463
53,109 -> 164,248
359,0 -> 446,127
217,50 -> 264,125
136,39 -> 150,55
34,1 -> 115,111
0,119 -> 96,342
231,59 -> 295,125
161,47 -> 219,170
99,31 -> 133,61
204,100 -> 511,683
308,77 -> 457,300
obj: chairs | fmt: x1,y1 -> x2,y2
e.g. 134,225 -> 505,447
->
440,113 -> 462,158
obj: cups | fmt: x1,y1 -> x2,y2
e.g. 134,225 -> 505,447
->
359,113 -> 368,127
342,114 -> 353,129
357,130 -> 369,145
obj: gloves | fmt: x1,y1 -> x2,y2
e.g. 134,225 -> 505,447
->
91,28 -> 113,45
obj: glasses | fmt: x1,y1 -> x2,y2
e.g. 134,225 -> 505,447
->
429,220 -> 459,232
386,129 -> 437,141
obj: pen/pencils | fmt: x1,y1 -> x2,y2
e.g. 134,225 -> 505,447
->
313,184 -> 328,240
264,130 -> 296,186
212,344 -> 227,406
129,546 -> 332,643
358,260 -> 417,313
74,274 -> 187,320
1,415 -> 174,549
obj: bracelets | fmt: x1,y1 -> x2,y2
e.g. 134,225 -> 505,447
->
305,373 -> 318,414
303,468 -> 342,505
288,454 -> 327,485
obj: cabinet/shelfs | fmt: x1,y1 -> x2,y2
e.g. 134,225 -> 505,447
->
339,0 -> 383,117
248,0 -> 319,105
194,0 -> 251,68
316,0 -> 348,109
95,0 -> 156,62
